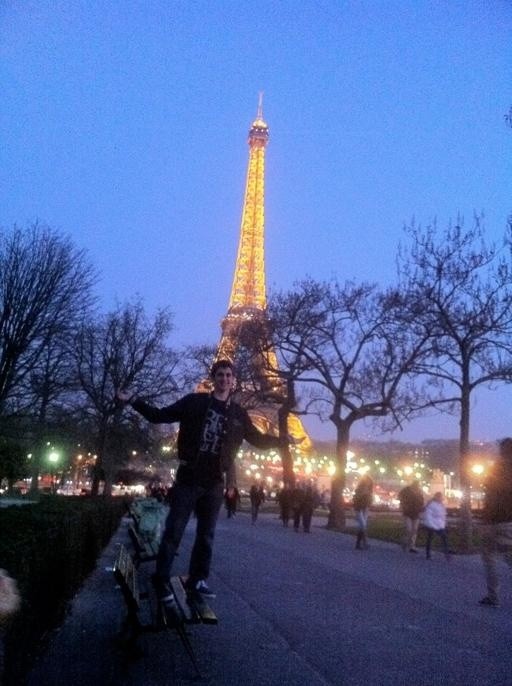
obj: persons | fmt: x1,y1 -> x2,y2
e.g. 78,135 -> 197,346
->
396,480 -> 427,553
114,360 -> 307,600
423,491 -> 452,560
475,438 -> 512,608
147,477 -> 331,534
351,474 -> 374,552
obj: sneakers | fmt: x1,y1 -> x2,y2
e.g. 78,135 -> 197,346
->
186,579 -> 216,599
155,581 -> 173,601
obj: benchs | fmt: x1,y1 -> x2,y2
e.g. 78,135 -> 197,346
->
112,542 -> 218,669
124,495 -> 179,567
